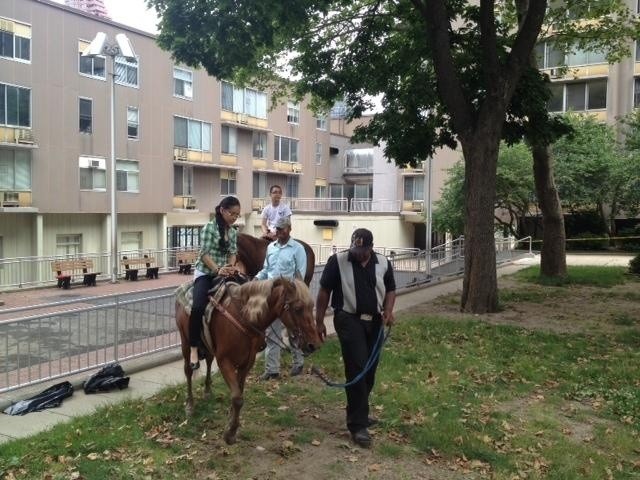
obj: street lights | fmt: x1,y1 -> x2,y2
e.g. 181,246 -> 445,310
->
82,28 -> 138,285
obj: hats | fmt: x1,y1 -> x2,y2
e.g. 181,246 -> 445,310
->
350,228 -> 375,249
273,217 -> 292,229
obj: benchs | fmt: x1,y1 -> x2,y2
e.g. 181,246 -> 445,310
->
119,254 -> 159,281
177,252 -> 198,275
51,259 -> 102,290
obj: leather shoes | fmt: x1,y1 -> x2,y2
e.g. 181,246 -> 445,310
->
256,371 -> 281,381
366,415 -> 379,426
352,426 -> 373,449
189,359 -> 200,371
289,364 -> 304,376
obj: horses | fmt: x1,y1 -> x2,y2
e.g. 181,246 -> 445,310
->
174,269 -> 323,446
233,232 -> 315,338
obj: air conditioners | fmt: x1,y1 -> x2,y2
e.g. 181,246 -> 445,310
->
89,159 -> 100,168
183,198 -> 197,207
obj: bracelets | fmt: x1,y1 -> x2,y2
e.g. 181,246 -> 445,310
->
265,230 -> 269,233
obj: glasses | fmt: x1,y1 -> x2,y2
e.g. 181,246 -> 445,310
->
220,207 -> 242,219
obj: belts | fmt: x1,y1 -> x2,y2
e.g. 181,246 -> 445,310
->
337,307 -> 379,322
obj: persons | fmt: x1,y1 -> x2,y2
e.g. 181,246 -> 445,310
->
189,197 -> 248,372
329,245 -> 339,256
258,186 -> 292,242
316,229 -> 396,448
251,217 -> 308,381
389,251 -> 396,269
